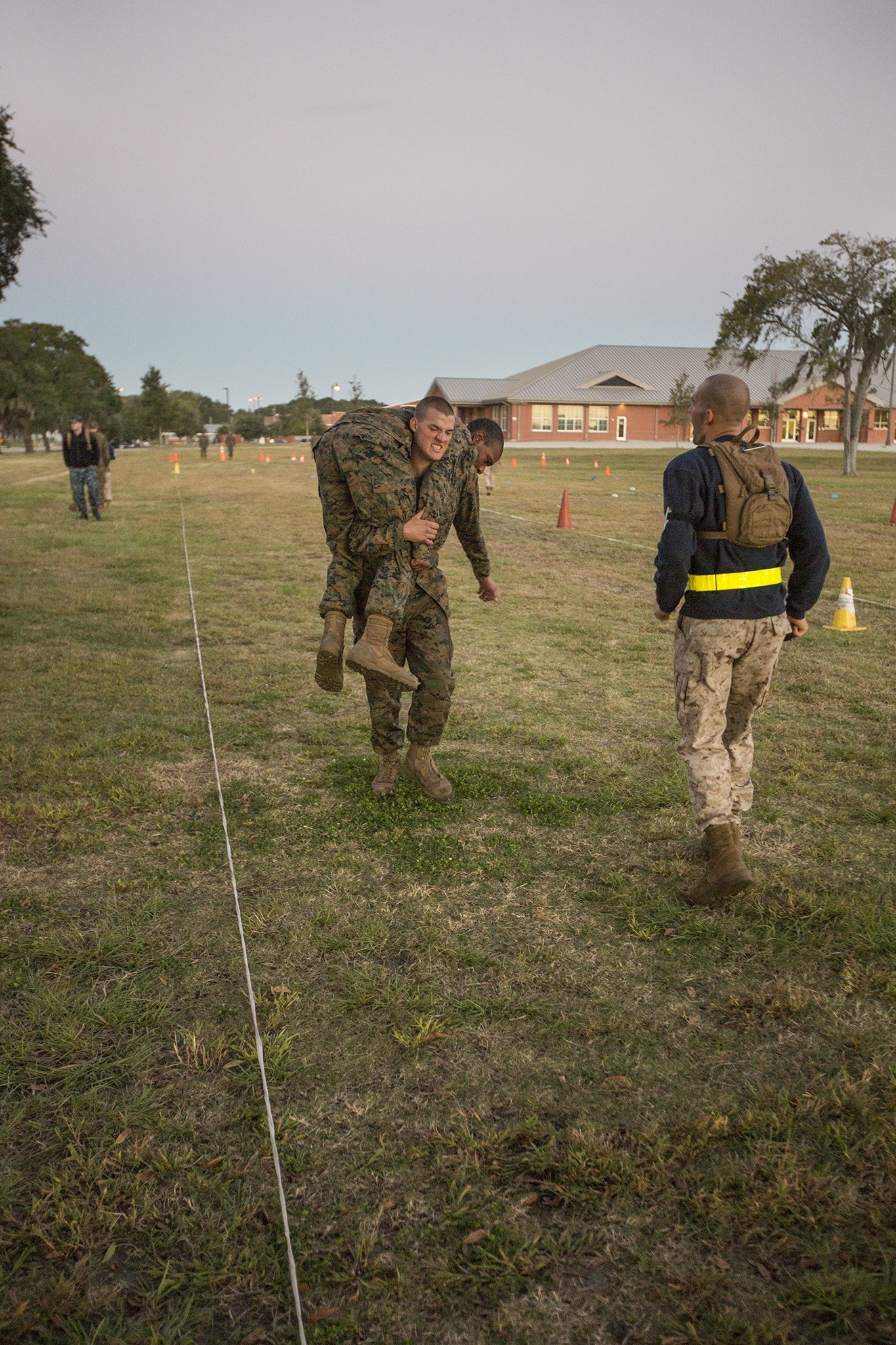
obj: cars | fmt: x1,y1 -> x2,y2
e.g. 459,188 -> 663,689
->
110,436 -> 151,449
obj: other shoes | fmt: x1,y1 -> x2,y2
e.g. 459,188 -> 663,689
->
93,510 -> 102,520
79,512 -> 89,520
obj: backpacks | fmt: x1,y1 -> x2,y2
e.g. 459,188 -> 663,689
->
697,424 -> 794,549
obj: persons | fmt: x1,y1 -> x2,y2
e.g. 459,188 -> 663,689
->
653,373 -> 831,902
353,395 -> 499,800
63,416 -> 102,520
68,420 -> 116,511
312,400 -> 504,692
199,431 -> 210,457
225,430 -> 235,456
483,460 -> 499,496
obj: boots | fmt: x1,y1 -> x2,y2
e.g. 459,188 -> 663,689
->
346,614 -> 419,692
701,823 -> 742,858
404,741 -> 455,802
314,610 -> 346,692
371,748 -> 402,795
691,824 -> 754,906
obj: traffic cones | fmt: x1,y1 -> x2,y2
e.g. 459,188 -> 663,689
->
604,465 -> 610,477
564,457 -> 570,466
299,453 -> 305,462
593,459 -> 599,468
219,445 -> 226,461
541,452 -> 547,467
168,452 -> 178,463
823,577 -> 868,633
265,454 -> 270,463
556,489 -> 573,529
173,462 -> 180,474
511,458 -> 516,467
259,453 -> 264,462
290,452 -> 297,461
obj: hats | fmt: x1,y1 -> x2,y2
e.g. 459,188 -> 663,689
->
68,415 -> 82,424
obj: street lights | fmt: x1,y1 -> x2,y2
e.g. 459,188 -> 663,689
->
223,387 -> 229,404
248,397 -> 262,411
331,382 -> 340,399
